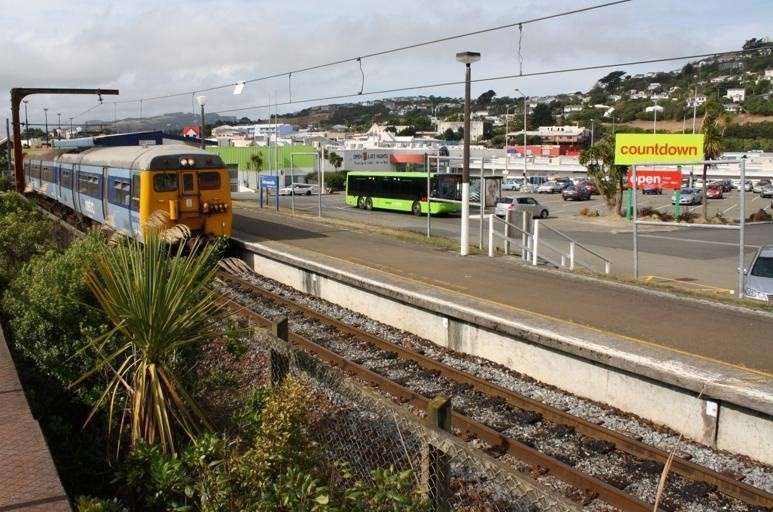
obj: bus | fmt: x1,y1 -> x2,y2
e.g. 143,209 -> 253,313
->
343,169 -> 470,222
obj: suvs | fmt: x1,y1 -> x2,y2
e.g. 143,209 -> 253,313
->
495,196 -> 549,220
278,183 -> 312,195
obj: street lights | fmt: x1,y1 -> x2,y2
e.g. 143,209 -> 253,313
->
42,107 -> 49,147
648,83 -> 698,135
197,95 -> 211,150
455,50 -> 485,258
513,86 -> 530,187
504,103 -> 520,186
56,112 -> 61,147
588,118 -> 597,153
23,99 -> 29,146
69,117 -> 73,140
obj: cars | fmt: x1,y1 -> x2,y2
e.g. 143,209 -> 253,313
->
641,183 -> 664,196
669,174 -> 773,205
502,171 -> 630,200
735,242 -> 773,305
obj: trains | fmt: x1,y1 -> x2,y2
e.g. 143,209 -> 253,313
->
12,120 -> 242,262
